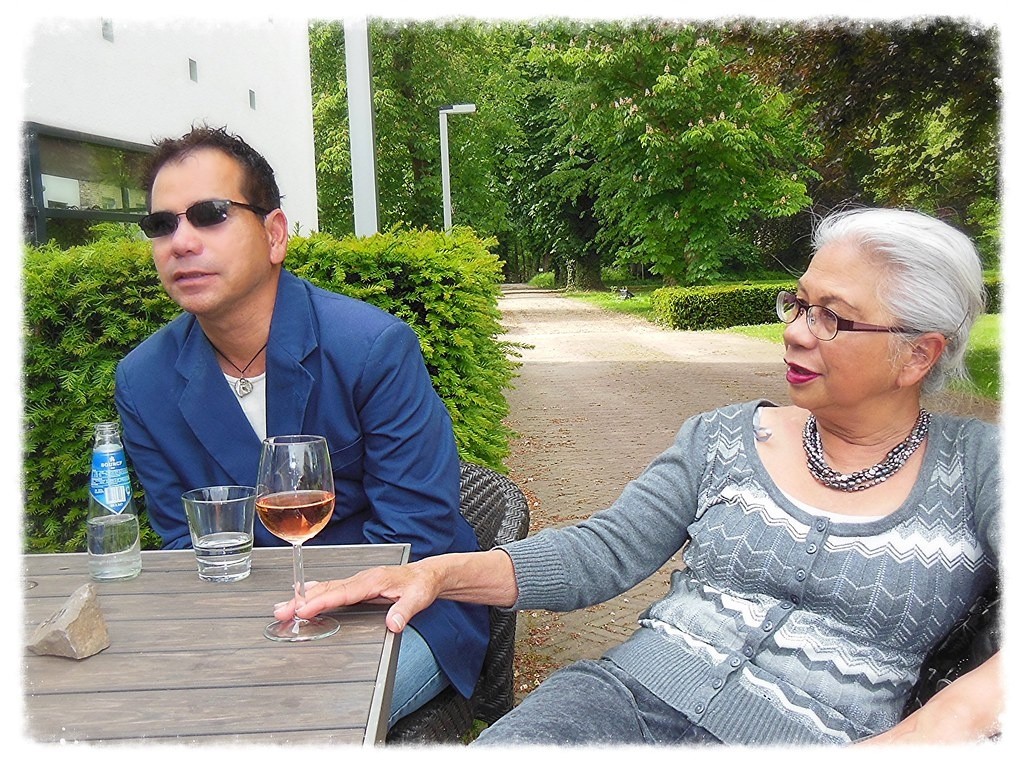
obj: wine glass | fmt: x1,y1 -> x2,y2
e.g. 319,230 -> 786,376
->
254,434 -> 341,643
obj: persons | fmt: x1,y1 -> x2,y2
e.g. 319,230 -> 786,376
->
117,121 -> 491,733
274,198 -> 1001,744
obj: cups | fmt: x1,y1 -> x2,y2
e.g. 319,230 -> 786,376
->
181,485 -> 257,584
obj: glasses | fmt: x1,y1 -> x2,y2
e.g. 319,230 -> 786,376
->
138,199 -> 271,238
775,291 -> 926,341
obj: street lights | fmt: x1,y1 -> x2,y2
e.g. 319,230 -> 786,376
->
438,102 -> 476,235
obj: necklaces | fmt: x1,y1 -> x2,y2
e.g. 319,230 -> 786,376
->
801,407 -> 931,491
204,334 -> 267,398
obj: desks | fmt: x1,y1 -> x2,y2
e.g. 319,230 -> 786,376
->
21,544 -> 409,745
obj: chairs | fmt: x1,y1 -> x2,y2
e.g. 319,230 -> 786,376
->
387,462 -> 530,746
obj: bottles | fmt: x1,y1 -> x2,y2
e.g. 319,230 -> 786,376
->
87,422 -> 144,583
